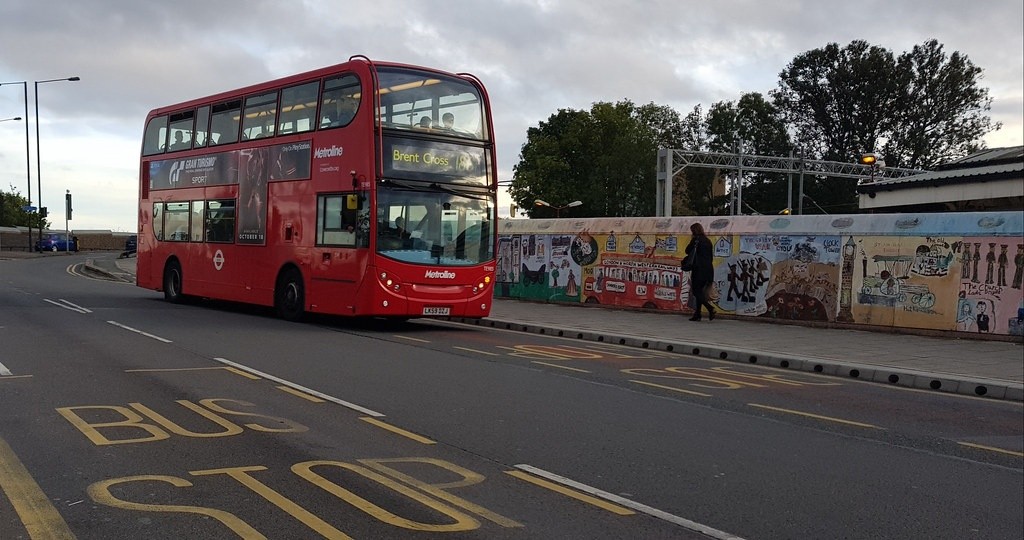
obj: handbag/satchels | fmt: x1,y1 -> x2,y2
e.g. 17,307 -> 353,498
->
703,282 -> 718,301
681,236 -> 699,270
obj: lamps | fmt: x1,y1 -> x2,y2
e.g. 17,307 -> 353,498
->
862,152 -> 876,183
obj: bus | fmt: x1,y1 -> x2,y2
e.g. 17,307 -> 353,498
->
133,55 -> 520,325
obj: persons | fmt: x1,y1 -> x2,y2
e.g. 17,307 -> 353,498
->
685,223 -> 717,321
330,97 -> 357,128
420,113 -> 454,130
394,217 -> 414,250
171,130 -> 188,151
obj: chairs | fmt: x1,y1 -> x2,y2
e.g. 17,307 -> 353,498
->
159,131 -> 284,151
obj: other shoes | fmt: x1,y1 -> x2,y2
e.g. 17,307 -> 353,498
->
709,310 -> 717,321
689,315 -> 702,321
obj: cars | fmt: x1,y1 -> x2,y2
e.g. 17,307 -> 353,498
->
124,234 -> 136,253
34,232 -> 76,253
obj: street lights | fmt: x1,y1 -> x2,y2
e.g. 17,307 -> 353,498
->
32,75 -> 80,252
533,199 -> 583,219
0,81 -> 33,252
